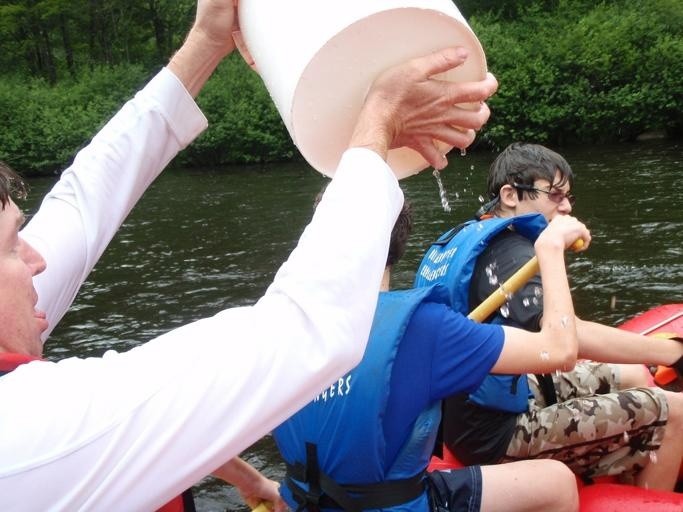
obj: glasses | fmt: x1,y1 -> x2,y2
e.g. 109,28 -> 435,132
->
526,187 -> 577,206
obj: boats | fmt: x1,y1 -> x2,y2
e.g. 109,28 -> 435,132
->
426,304 -> 683,512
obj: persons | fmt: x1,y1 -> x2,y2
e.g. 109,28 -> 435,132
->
0,1 -> 500,512
413,137 -> 681,493
267,179 -> 594,512
213,458 -> 287,512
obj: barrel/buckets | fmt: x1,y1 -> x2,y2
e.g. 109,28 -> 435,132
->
235,0 -> 490,182
